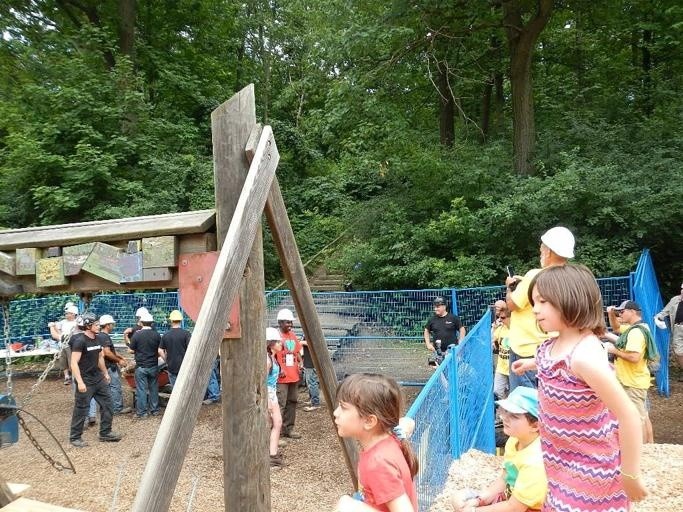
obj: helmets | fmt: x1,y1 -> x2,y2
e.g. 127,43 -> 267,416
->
264,326 -> 285,341
540,225 -> 578,262
277,308 -> 297,323
63,302 -> 117,329
134,306 -> 156,323
168,309 -> 184,322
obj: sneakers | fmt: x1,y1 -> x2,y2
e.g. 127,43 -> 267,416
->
87,406 -> 161,426
63,376 -> 73,385
269,400 -> 322,468
203,395 -> 220,405
98,430 -> 123,442
71,436 -> 89,448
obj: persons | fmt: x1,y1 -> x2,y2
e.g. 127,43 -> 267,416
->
159,310 -> 222,404
265,308 -> 320,466
422,297 -> 467,403
48,302 -> 127,447
333,375 -> 418,512
488,226 -> 653,512
124,306 -> 162,418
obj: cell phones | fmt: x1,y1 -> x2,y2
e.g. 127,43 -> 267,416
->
507,265 -> 514,277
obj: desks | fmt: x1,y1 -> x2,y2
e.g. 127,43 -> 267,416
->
0,349 -> 57,359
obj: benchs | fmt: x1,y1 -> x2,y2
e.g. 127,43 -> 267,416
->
121,386 -> 171,411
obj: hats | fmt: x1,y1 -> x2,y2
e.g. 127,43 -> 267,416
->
491,383 -> 541,421
611,299 -> 641,313
